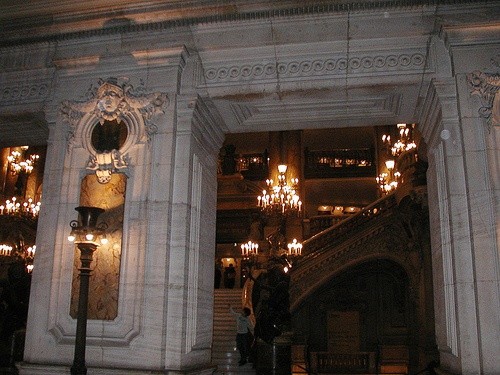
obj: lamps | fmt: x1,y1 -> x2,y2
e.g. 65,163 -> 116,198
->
68,206 -> 109,245
383,155 -> 395,180
276,161 -> 288,186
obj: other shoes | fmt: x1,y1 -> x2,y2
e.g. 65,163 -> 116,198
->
238,360 -> 247,366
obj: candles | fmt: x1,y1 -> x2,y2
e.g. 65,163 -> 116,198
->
287,239 -> 303,255
27,245 -> 36,257
7,150 -> 39,175
267,158 -> 270,167
241,241 -> 259,255
27,265 -> 34,273
257,177 -> 302,213
0,197 -> 41,217
376,172 -> 401,195
381,128 -> 416,156
0,244 -> 12,256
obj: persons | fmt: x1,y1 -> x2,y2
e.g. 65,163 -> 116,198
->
223,263 -> 236,289
226,307 -> 251,366
0,260 -> 32,362
215,262 -> 221,289
